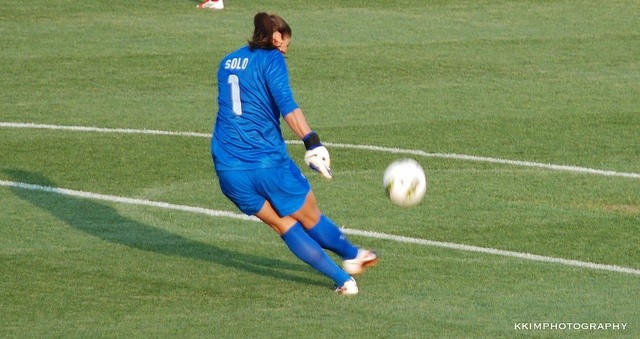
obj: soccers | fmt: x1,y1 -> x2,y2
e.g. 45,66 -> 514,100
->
384,158 -> 427,207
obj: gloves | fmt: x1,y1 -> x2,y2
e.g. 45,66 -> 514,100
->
302,132 -> 330,178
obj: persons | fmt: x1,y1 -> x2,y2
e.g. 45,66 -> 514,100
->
210,12 -> 379,295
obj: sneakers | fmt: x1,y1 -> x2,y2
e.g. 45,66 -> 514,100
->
342,249 -> 377,276
335,276 -> 358,295
